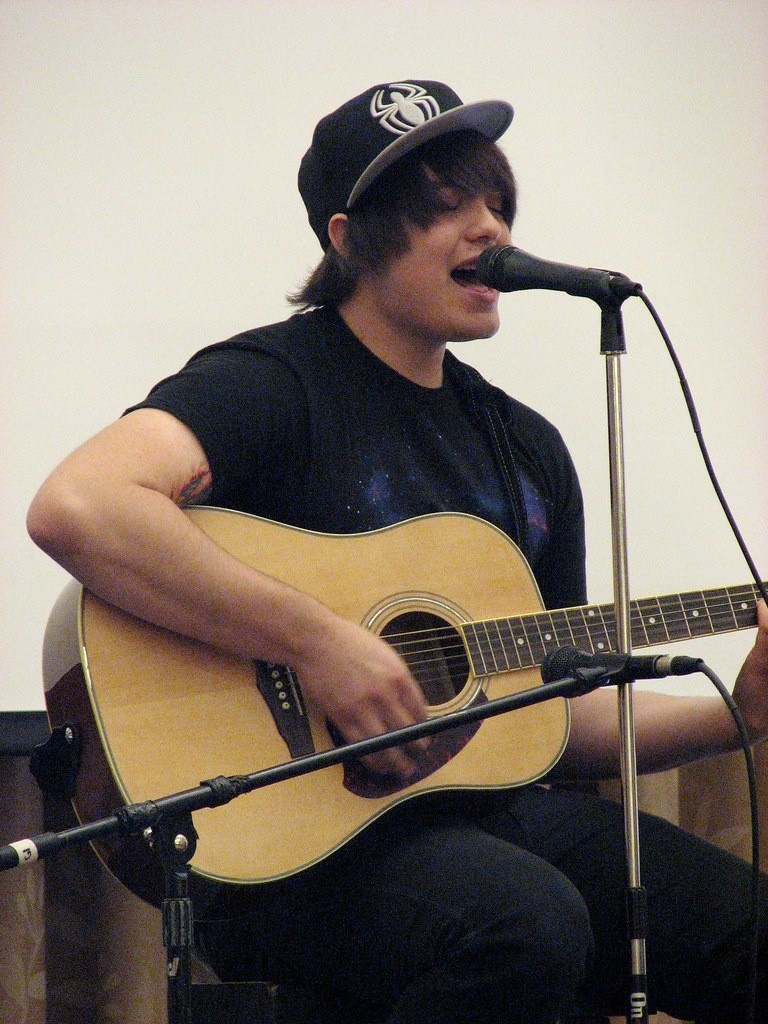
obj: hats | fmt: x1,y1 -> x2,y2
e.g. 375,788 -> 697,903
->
297,79 -> 515,247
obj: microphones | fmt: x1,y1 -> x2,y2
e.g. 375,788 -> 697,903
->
540,645 -> 705,698
476,244 -> 642,297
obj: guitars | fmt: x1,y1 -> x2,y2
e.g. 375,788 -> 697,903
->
29,494 -> 768,931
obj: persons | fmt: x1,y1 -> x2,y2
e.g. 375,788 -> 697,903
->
25,78 -> 768,1024
